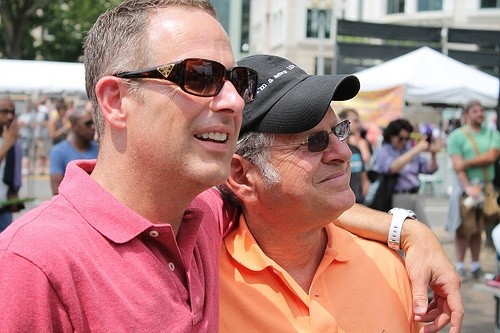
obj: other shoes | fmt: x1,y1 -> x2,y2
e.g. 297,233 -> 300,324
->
471,269 -> 495,281
457,268 -> 469,282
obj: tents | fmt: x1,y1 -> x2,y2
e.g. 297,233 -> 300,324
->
342,44 -> 500,108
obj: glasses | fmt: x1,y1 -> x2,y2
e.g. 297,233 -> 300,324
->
0,108 -> 16,114
250,119 -> 351,153
398,135 -> 411,142
84,119 -> 95,127
112,57 -> 259,103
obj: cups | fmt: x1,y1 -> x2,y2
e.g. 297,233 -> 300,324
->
463,192 -> 485,209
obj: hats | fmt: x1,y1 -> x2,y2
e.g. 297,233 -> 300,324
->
236,54 -> 360,134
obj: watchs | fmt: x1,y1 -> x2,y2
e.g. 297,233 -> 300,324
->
388,208 -> 418,251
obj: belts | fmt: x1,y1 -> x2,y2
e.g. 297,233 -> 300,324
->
395,188 -> 420,193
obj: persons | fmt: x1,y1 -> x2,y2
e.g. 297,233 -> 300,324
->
9,94 -> 96,179
448,99 -> 500,283
205,55 -> 424,333
375,120 -> 441,304
0,0 -> 466,333
445,105 -> 499,136
51,109 -> 102,195
0,93 -> 25,234
338,108 -> 372,202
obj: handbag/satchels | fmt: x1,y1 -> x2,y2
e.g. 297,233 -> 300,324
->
363,170 -> 399,211
482,181 -> 500,215
